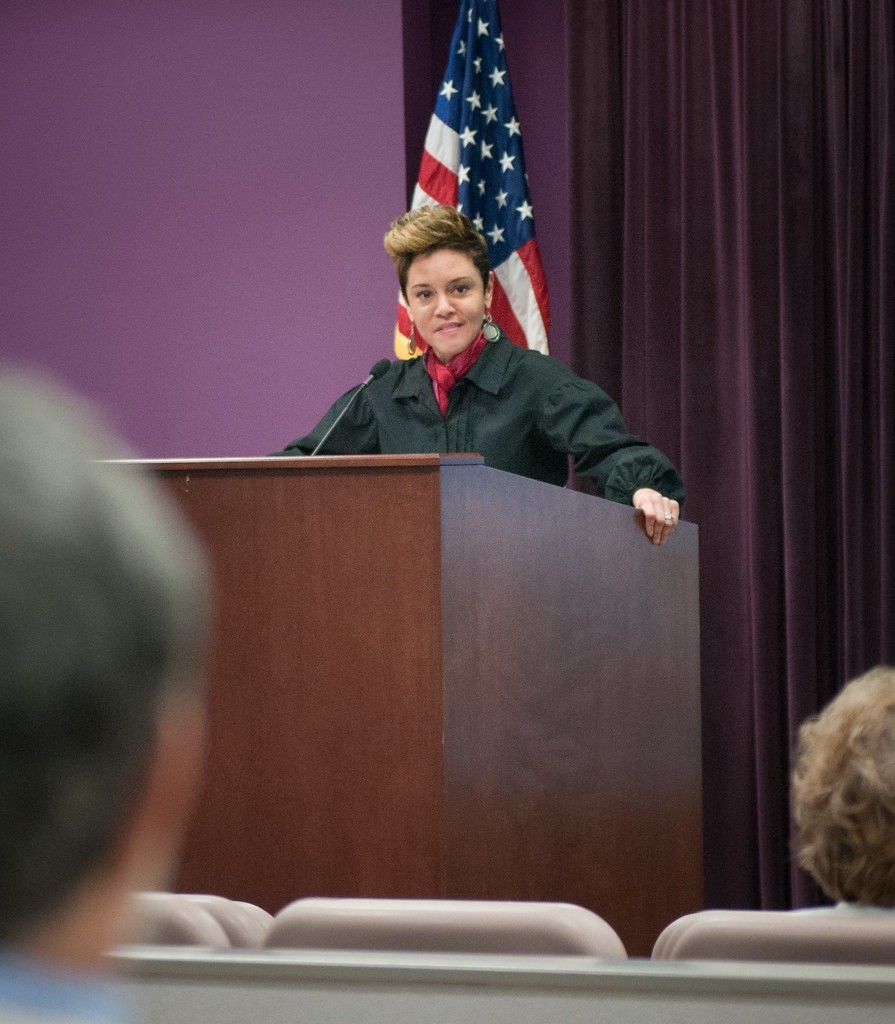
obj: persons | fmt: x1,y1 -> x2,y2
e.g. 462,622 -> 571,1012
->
266,203 -> 686,545
0,362 -> 219,1024
790,665 -> 895,920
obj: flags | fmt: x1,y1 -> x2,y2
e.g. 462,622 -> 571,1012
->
392,0 -> 553,362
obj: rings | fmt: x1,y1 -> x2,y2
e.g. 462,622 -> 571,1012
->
665,512 -> 675,520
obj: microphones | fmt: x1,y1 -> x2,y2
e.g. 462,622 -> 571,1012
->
309,358 -> 391,456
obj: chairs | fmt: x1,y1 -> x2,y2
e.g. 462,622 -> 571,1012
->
117,888 -> 895,961
